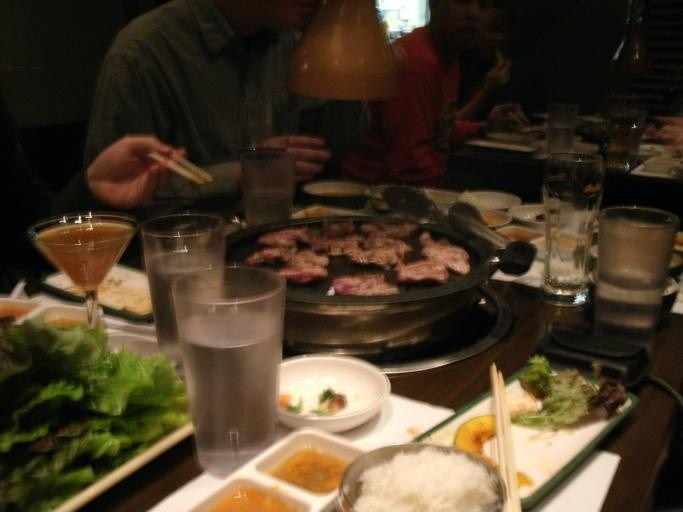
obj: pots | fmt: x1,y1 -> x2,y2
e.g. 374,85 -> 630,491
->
214,211 -> 534,356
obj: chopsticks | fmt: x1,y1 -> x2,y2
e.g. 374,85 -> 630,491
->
487,360 -> 523,512
148,144 -> 214,187
509,106 -> 535,131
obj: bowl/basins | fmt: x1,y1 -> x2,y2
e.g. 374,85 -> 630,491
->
589,227 -> 683,330
272,350 -> 392,432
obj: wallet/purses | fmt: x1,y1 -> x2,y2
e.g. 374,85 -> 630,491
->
536,326 -> 652,389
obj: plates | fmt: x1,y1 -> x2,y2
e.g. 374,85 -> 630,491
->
184,426 -> 368,512
291,179 -> 547,263
1,295 -> 104,342
461,124 -> 682,182
52,326 -> 195,512
45,257 -> 161,321
414,368 -> 646,508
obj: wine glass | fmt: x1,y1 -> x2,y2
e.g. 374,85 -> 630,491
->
26,212 -> 134,329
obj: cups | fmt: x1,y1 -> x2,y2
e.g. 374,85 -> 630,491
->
594,206 -> 677,352
238,146 -> 298,226
141,208 -> 230,362
172,264 -> 288,478
541,85 -> 646,172
542,149 -> 605,306
